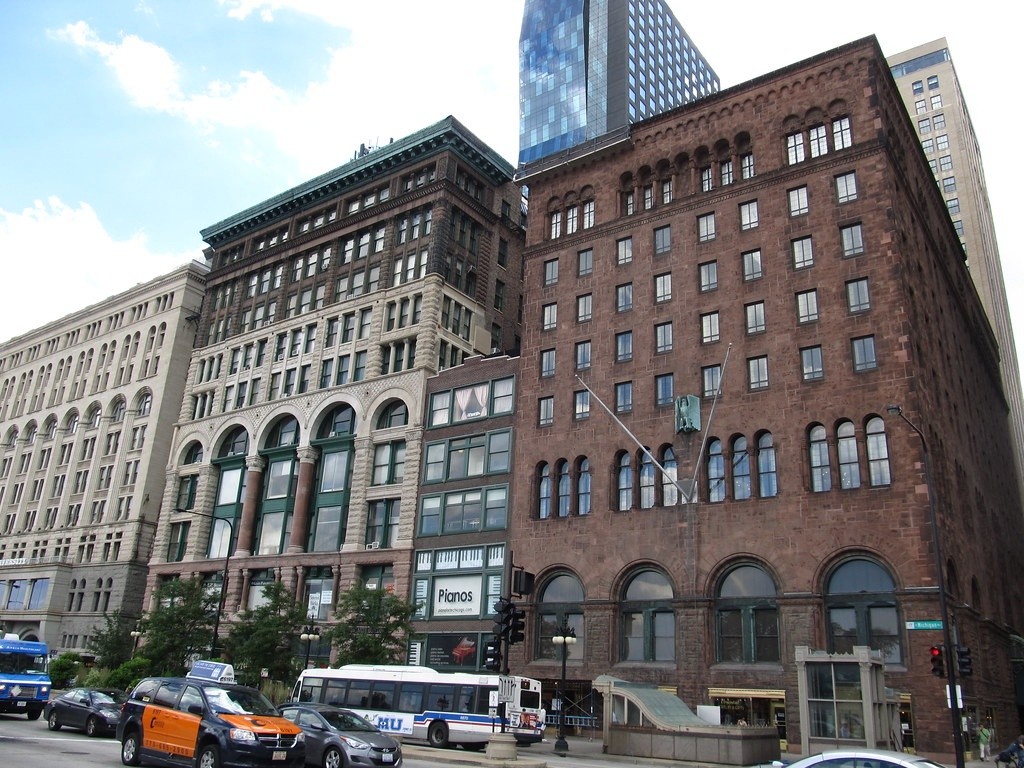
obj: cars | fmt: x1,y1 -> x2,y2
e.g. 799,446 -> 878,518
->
274,701 -> 403,768
43,687 -> 130,737
749,748 -> 948,768
116,660 -> 307,768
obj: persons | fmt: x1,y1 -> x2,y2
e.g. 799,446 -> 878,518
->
840,723 -> 848,737
995,735 -> 1024,768
737,715 -> 747,726
976,723 -> 991,762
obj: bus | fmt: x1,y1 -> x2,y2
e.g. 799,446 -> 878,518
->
288,663 -> 521,752
0,632 -> 52,720
502,675 -> 546,748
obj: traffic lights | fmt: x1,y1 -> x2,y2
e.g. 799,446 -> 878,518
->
957,645 -> 973,676
507,604 -> 526,645
484,641 -> 501,671
524,572 -> 535,595
929,645 -> 944,677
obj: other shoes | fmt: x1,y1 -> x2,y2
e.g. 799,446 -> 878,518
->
980,757 -> 984,761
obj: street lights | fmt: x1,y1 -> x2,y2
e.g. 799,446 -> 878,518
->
130,619 -> 145,659
552,625 -> 577,752
300,614 -> 320,669
172,507 -> 234,659
